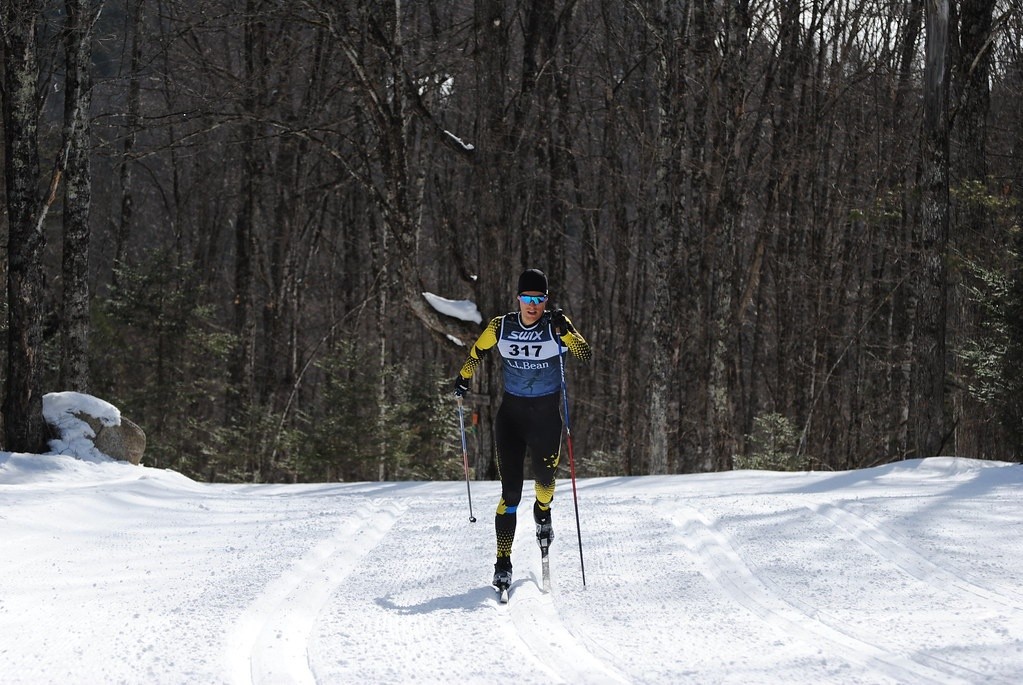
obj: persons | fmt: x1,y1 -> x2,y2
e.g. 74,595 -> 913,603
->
454,269 -> 593,593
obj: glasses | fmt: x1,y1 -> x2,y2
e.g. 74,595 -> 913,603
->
519,294 -> 547,305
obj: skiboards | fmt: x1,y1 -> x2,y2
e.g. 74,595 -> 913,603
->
498,550 -> 552,604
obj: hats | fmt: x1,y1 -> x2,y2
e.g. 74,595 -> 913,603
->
517,268 -> 548,295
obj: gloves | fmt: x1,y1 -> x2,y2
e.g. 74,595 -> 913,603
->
549,308 -> 568,335
453,375 -> 469,399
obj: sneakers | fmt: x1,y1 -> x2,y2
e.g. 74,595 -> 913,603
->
533,501 -> 554,549
493,555 -> 513,589
